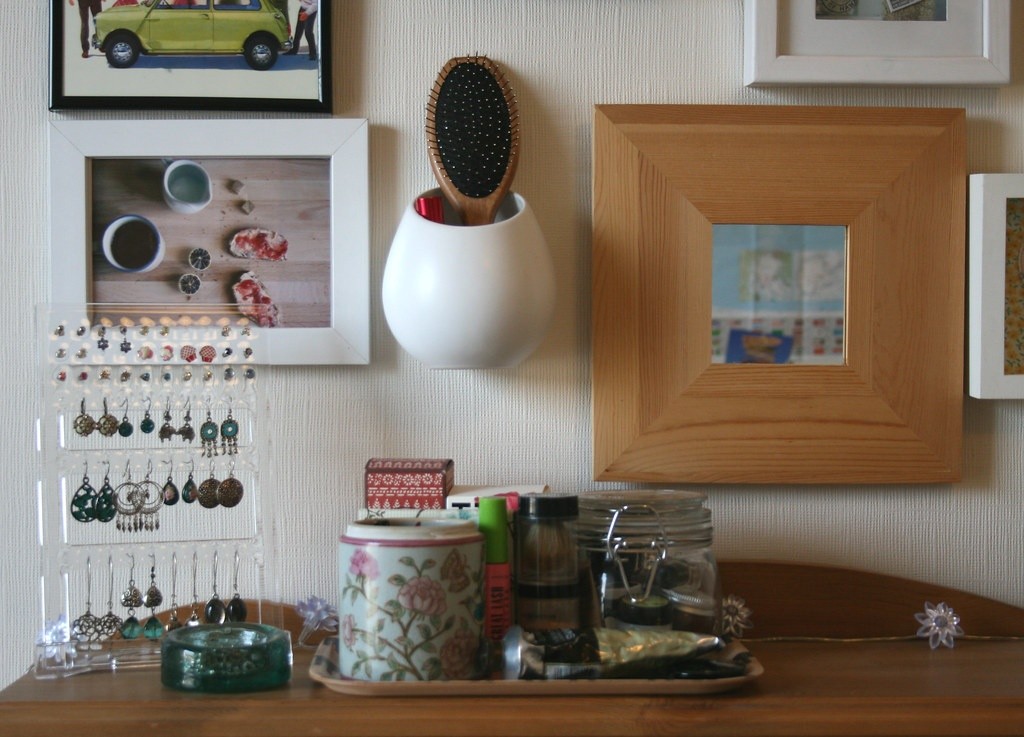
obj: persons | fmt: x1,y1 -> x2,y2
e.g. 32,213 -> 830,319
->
280,0 -> 318,61
69,0 -> 104,58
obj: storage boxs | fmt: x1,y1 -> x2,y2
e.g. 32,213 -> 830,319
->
363,457 -> 546,510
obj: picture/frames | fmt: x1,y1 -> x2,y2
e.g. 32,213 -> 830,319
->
47,0 -> 332,114
967,172 -> 1024,400
48,120 -> 371,365
744,0 -> 1012,88
589,103 -> 967,485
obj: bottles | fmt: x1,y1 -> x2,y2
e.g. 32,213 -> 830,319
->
380,188 -> 555,371
516,492 -> 580,631
568,482 -> 715,639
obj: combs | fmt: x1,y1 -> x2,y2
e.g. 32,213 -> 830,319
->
425,50 -> 521,225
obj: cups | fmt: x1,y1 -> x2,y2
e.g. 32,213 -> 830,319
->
102,215 -> 166,274
160,158 -> 212,214
336,516 -> 484,682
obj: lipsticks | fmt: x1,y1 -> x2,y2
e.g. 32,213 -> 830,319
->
416,196 -> 446,225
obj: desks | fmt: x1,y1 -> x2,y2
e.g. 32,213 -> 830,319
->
1,559 -> 1024,736
